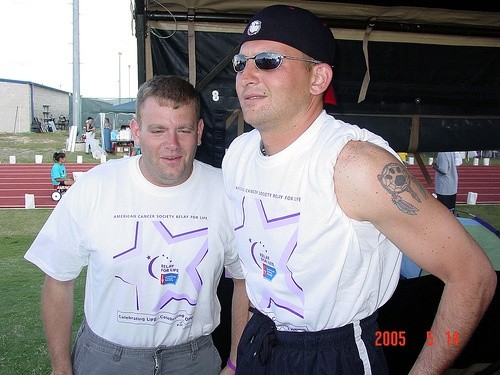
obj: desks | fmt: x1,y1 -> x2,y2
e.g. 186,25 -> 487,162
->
112,138 -> 134,151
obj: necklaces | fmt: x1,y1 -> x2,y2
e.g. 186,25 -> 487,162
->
261,145 -> 271,157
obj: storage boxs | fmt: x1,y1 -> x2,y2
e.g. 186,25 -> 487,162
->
114,143 -> 141,156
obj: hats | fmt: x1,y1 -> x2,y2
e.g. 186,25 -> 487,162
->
238,3 -> 336,64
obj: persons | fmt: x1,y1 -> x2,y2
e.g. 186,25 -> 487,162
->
50,152 -> 74,188
219,4 -> 497,375
103,118 -> 113,153
24,76 -> 254,375
85,116 -> 95,154
431,151 -> 458,210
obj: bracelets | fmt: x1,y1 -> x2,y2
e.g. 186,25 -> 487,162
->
226,358 -> 236,371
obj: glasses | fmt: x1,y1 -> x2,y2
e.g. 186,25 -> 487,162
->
231,52 -> 322,73
